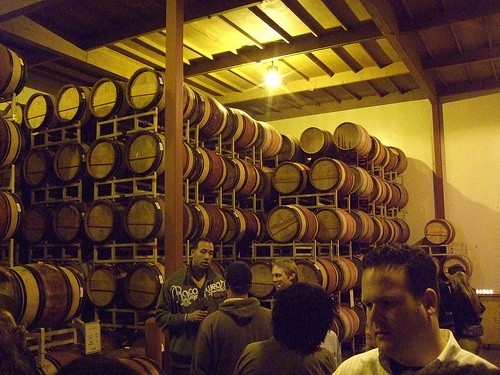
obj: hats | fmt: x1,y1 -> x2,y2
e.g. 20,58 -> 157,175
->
226,261 -> 253,288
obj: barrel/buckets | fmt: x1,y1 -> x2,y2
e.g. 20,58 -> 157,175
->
443,254 -> 473,279
1,43 -> 410,375
424,219 -> 456,245
432,257 -> 441,275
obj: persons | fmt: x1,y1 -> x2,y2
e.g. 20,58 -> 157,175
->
272,258 -> 298,292
190,260 -> 273,375
332,243 -> 500,375
153,238 -> 226,375
450,272 -> 486,354
233,282 -> 337,375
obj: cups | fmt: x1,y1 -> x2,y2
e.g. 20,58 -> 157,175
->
199,298 -> 208,311
